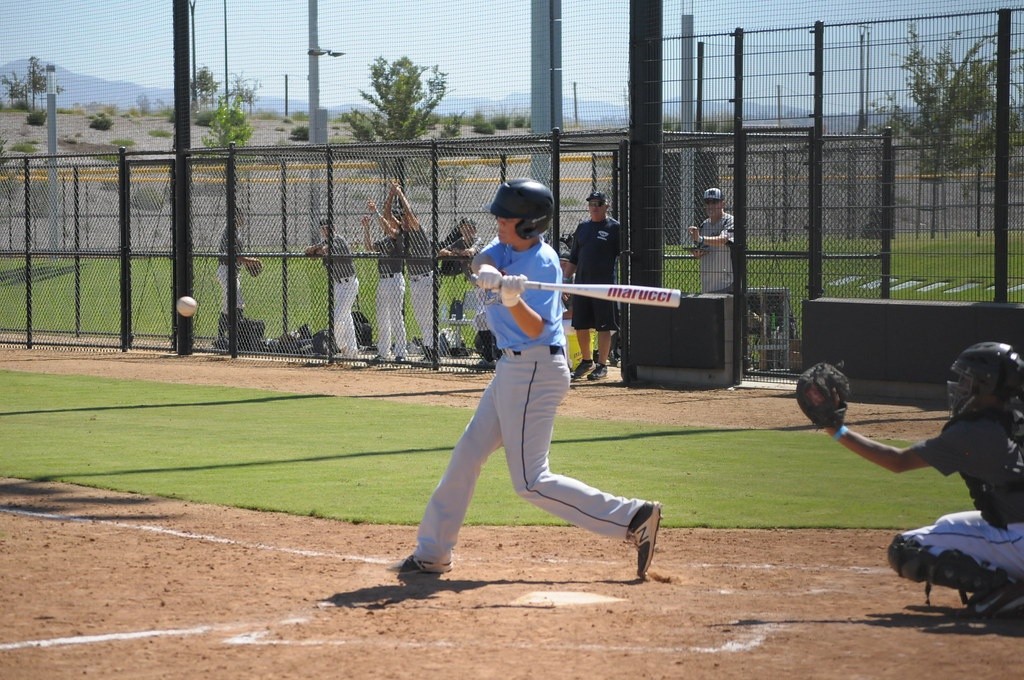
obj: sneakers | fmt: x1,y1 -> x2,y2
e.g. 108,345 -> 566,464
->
957,580 -> 1024,620
569,359 -> 597,381
394,554 -> 457,575
587,363 -> 609,379
629,502 -> 663,578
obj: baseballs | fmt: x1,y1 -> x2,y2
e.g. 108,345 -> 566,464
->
177,296 -> 198,318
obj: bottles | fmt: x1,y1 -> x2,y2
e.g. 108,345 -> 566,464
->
442,302 -> 447,321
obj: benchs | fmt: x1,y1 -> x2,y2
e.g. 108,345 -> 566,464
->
448,318 -> 475,348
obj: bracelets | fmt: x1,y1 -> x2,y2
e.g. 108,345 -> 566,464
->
563,278 -> 568,281
699,236 -> 703,243
833,426 -> 848,441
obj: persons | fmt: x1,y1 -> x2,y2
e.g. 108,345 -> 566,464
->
304,217 -> 359,362
387,177 -> 662,575
217,207 -> 261,340
384,181 -> 440,361
560,192 -> 621,381
796,342 -> 1024,617
361,198 -> 410,364
437,217 -> 501,368
687,188 -> 734,293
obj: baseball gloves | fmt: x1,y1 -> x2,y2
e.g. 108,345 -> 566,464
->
242,259 -> 266,278
796,360 -> 854,432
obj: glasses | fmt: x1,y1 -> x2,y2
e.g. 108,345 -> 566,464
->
589,201 -> 606,208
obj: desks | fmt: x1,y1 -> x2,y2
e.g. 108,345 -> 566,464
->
746,288 -> 790,371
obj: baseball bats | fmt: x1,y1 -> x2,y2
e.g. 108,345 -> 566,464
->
445,236 -> 465,251
468,271 -> 683,311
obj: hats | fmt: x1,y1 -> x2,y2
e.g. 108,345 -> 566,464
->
703,188 -> 724,203
585,192 -> 605,202
459,217 -> 477,228
319,218 -> 335,230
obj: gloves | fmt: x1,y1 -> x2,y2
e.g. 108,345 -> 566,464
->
475,264 -> 504,293
490,273 -> 528,307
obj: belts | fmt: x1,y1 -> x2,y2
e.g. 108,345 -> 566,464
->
513,344 -> 565,357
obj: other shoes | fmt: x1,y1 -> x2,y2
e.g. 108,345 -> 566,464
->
469,358 -> 497,370
369,354 -> 390,367
395,354 -> 405,364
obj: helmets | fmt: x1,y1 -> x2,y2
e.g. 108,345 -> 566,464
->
947,343 -> 1024,420
483,178 -> 553,240
